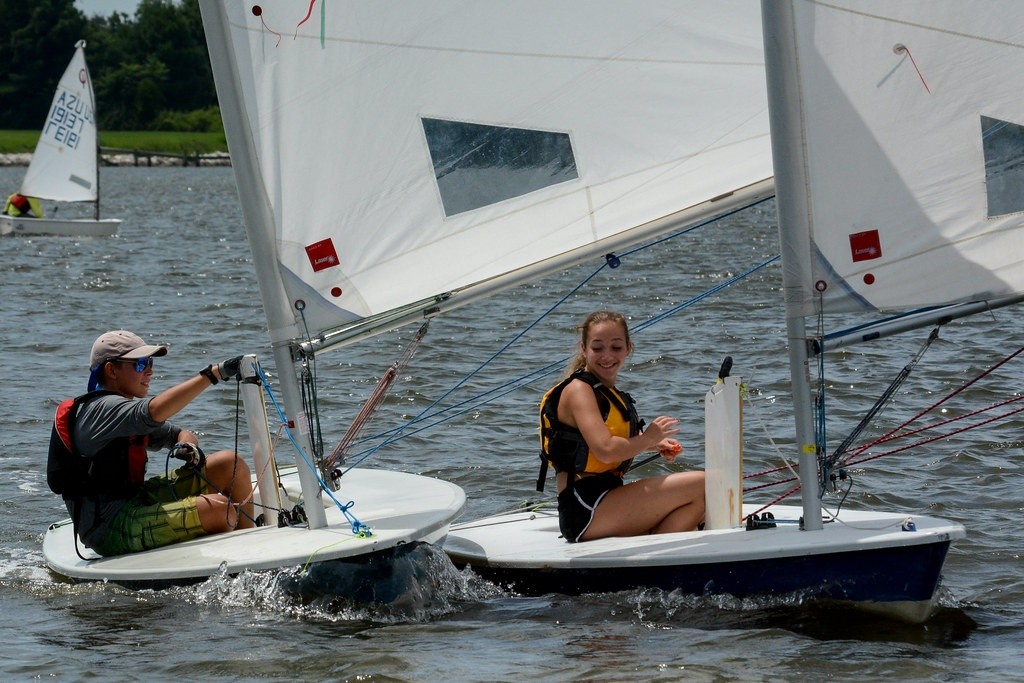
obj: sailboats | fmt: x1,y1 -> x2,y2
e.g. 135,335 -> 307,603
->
36,0 -> 781,592
0,40 -> 123,241
398,1 -> 1021,612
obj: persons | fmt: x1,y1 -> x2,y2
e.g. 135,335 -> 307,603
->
1,190 -> 47,219
61,328 -> 255,558
551,310 -> 706,544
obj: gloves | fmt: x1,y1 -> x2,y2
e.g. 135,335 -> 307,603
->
217,354 -> 244,381
175,443 -> 207,476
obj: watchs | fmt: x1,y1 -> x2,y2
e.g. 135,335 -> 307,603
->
198,364 -> 220,385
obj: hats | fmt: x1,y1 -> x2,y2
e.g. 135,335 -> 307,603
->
92,330 -> 168,365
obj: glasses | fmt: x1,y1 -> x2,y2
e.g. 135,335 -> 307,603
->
110,358 -> 154,371
